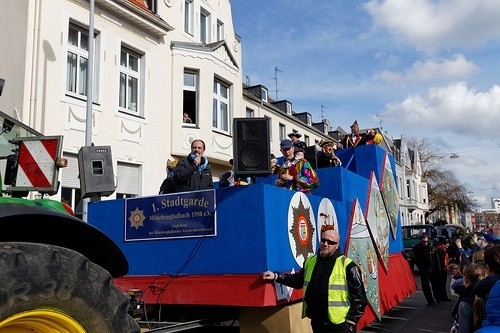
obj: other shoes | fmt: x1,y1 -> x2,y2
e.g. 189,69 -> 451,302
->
440,298 -> 452,302
427,301 -> 440,306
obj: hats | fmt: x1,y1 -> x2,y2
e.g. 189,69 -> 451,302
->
280,139 -> 293,150
166,156 -> 177,168
287,129 -> 302,138
318,139 -> 334,147
350,120 -> 359,129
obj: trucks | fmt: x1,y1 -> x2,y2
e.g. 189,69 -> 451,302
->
401,222 -> 467,273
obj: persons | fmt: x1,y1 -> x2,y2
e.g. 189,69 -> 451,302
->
274,139 -> 320,195
159,159 -> 179,195
263,154 -> 280,178
287,129 -> 307,159
412,213 -> 500,333
261,229 -> 368,333
219,159 -> 252,187
335,120 -> 378,148
317,138 -> 342,168
294,147 -> 305,159
172,139 -> 215,193
183,113 -> 192,123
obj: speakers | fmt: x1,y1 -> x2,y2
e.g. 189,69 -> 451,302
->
233,118 -> 272,180
77,146 -> 115,199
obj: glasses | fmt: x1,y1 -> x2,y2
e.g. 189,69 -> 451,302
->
321,238 -> 338,245
422,240 -> 428,243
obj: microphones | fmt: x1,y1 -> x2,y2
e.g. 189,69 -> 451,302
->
196,153 -> 200,167
